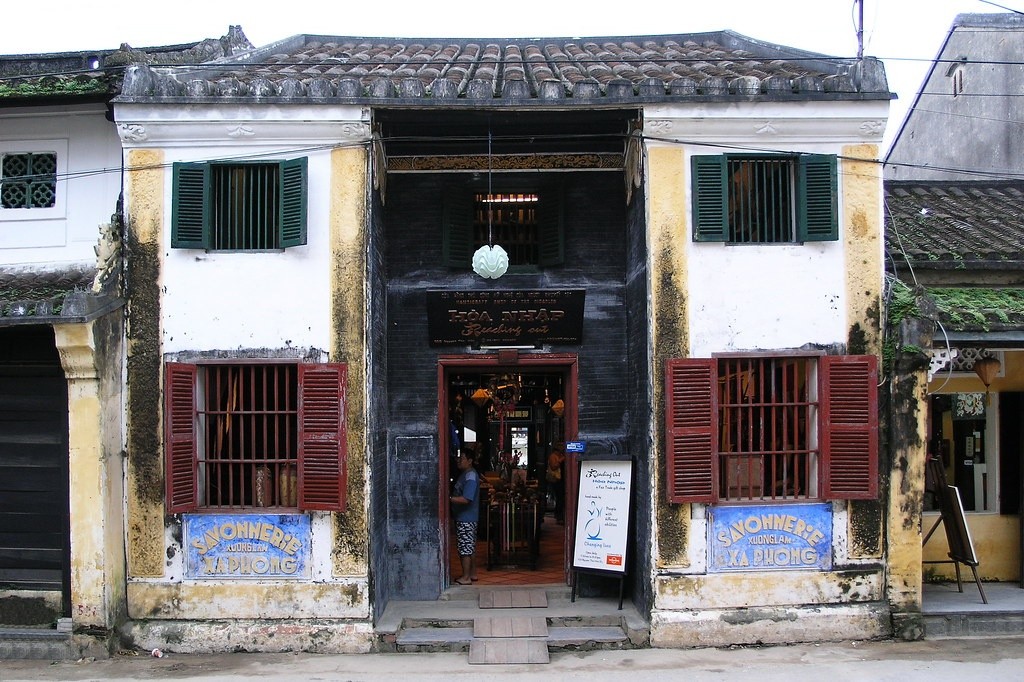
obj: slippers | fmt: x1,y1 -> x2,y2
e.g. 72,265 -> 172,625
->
470,578 -> 478,581
451,579 -> 471,586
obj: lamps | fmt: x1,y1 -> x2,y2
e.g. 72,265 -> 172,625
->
472,120 -> 510,279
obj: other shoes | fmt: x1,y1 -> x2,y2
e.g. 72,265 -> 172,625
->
556,519 -> 563,525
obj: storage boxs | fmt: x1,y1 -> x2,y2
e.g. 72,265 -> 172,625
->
730,455 -> 761,496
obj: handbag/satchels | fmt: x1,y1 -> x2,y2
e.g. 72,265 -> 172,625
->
545,458 -> 562,482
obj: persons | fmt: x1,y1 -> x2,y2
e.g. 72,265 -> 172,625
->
449,448 -> 481,585
548,441 -> 565,526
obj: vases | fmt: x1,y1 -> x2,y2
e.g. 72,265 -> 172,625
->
255,463 -> 296,507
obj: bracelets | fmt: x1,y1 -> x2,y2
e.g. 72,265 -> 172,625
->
449,496 -> 453,502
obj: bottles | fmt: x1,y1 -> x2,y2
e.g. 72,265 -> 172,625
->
543,388 -> 552,404
